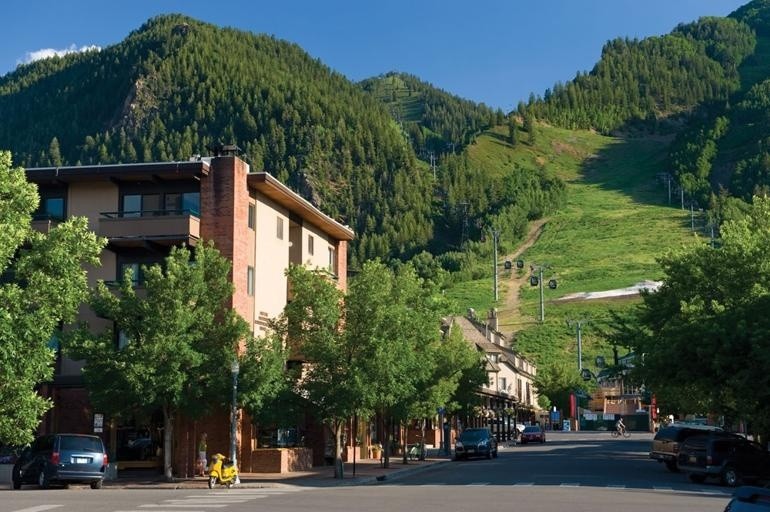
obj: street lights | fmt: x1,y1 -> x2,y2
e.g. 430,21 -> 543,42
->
230,359 -> 240,483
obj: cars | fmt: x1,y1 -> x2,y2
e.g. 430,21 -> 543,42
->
521,426 -> 546,444
649,417 -> 770,512
455,427 -> 498,460
482,418 -> 525,437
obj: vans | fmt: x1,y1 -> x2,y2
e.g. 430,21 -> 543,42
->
12,432 -> 109,489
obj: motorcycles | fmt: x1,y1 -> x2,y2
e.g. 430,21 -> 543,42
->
206,452 -> 238,489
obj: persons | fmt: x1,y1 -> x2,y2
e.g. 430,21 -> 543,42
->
615,418 -> 626,435
197,431 -> 209,476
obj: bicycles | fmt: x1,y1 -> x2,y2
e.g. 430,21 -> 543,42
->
611,427 -> 631,438
409,440 -> 428,461
